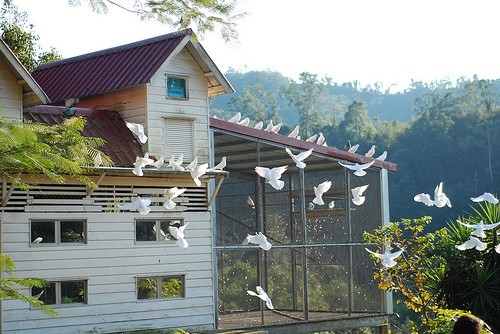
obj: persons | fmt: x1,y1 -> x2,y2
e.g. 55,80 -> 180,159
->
453,315 -> 480,334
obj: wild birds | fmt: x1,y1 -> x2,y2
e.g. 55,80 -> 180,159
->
469,191 -> 500,205
115,195 -> 152,215
162,185 -> 187,210
364,246 -> 404,268
246,195 -> 256,209
411,181 -> 453,209
32,237 -> 43,244
350,183 -> 370,206
130,152 -> 227,187
246,285 -> 275,310
285,147 -> 314,169
61,95 -> 80,117
337,160 -> 375,177
343,140 -> 388,161
125,120 -> 149,145
254,165 -> 289,191
226,111 -> 328,147
167,220 -> 190,249
240,231 -> 273,251
454,219 -> 500,254
306,179 -> 336,211
